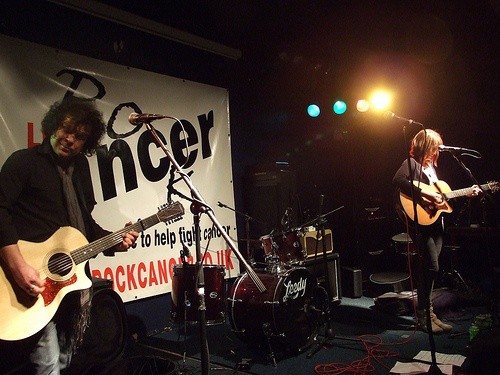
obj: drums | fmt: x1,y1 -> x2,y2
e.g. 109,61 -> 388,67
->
227,265 -> 320,343
260,228 -> 305,266
392,232 -> 417,256
169,264 -> 226,326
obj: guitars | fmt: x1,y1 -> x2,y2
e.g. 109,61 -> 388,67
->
393,179 -> 500,230
0,199 -> 185,341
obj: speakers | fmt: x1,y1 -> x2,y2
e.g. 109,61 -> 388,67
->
300,229 -> 333,258
302,252 -> 342,304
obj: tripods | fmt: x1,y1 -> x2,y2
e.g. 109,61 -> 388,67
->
272,206 -> 377,360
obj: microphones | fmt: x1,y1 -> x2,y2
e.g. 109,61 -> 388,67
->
385,110 -> 418,125
129,112 -> 165,125
281,207 -> 292,226
438,145 -> 482,159
181,241 -> 191,258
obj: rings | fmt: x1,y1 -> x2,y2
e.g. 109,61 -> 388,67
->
31,285 -> 35,290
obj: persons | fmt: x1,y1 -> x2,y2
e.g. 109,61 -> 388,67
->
0,96 -> 140,375
392,129 -> 483,333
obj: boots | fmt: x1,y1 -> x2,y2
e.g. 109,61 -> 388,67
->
417,309 -> 443,334
429,307 -> 453,332
